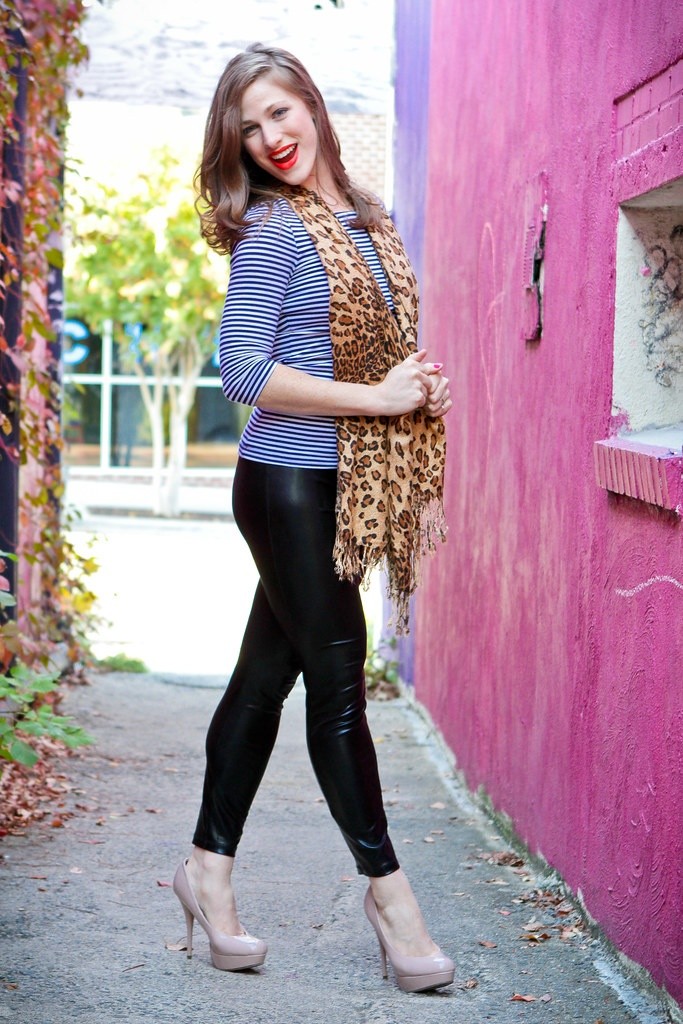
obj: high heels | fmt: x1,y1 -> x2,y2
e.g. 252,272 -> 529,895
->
364,882 -> 454,994
173,855 -> 267,971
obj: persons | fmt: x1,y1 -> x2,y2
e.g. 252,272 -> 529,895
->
174,44 -> 454,994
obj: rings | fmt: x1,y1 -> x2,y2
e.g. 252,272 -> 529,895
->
441,406 -> 446,414
440,399 -> 445,405
